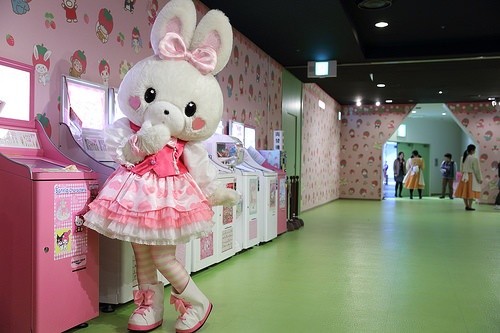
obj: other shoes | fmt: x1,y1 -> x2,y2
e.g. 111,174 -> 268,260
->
495,204 -> 500,209
450,196 -> 454,199
466,206 -> 475,210
439,196 -> 445,198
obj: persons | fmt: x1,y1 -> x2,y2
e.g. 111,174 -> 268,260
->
402,150 -> 426,199
495,162 -> 500,209
439,153 -> 457,199
393,152 -> 406,197
454,144 -> 483,210
383,161 -> 388,185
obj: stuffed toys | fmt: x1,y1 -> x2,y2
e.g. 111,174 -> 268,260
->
83,0 -> 242,333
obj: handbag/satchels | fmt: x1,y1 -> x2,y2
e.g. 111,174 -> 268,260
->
472,157 -> 482,192
